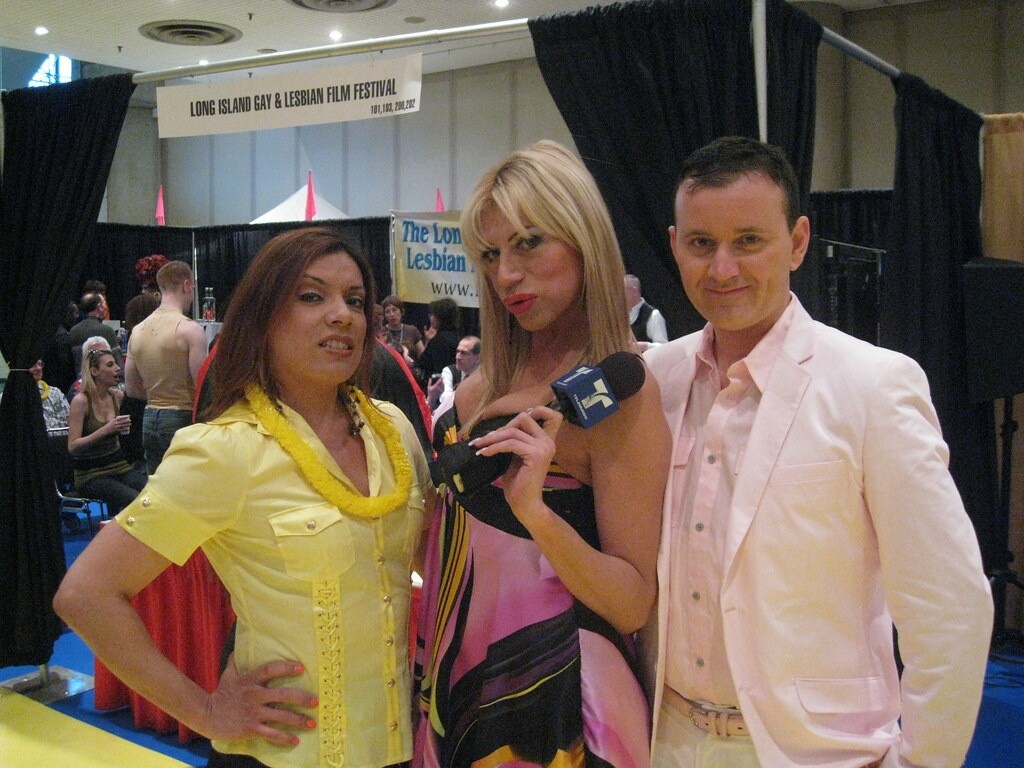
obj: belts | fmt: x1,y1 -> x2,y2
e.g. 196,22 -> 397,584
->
662,684 -> 750,738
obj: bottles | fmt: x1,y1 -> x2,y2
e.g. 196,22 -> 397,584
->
202,287 -> 215,322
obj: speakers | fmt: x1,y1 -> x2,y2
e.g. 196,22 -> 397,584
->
960,255 -> 1024,403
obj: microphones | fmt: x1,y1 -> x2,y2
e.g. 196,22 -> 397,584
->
437,353 -> 646,497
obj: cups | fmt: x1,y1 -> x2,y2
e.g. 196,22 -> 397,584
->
116,415 -> 130,434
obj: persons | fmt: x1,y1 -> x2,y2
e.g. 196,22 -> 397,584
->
371,294 -> 482,424
411,139 -> 674,768
621,274 -> 669,353
631,135 -> 995,768
51,228 -> 435,768
26,255 -> 221,509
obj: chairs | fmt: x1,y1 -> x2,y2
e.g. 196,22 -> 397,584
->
47,427 -> 107,539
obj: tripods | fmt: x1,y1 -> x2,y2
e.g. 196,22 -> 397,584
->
985,396 -> 1024,646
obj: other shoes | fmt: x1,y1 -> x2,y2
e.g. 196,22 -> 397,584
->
63,513 -> 82,532
